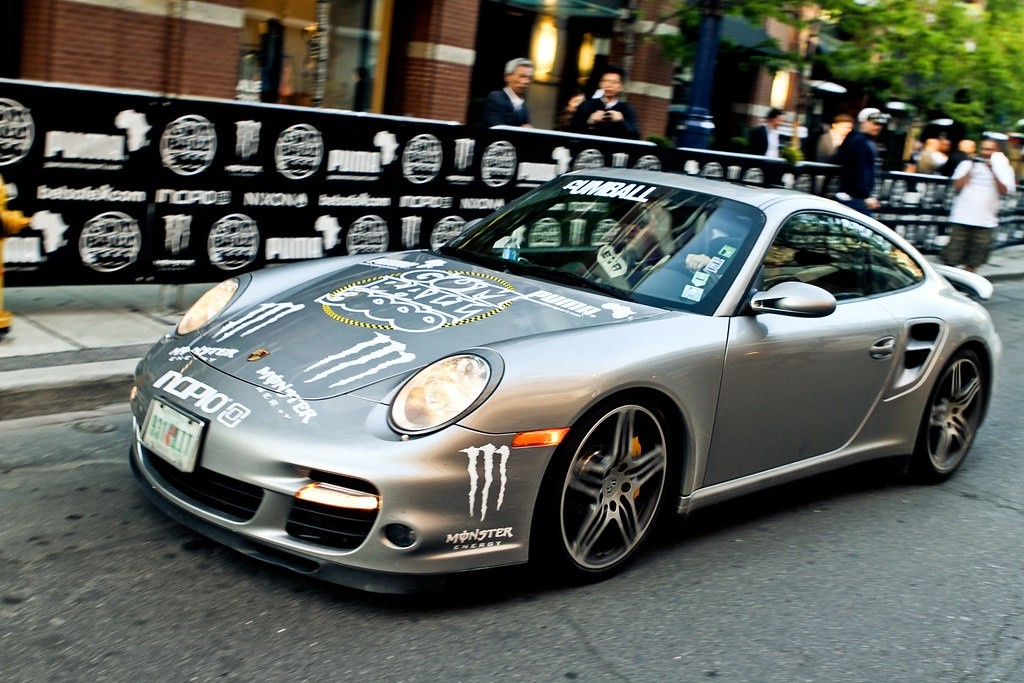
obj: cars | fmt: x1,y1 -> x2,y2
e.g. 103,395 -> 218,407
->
127,164 -> 1003,601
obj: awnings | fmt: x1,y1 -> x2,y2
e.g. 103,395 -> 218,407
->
718,18 -> 786,59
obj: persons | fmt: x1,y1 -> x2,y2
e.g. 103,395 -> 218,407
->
685,210 -> 764,307
479,59 -> 532,128
560,68 -> 641,141
747,107 -> 785,159
591,202 -> 677,291
814,107 -> 1024,273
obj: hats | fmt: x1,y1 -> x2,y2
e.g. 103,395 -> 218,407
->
858,108 -> 887,124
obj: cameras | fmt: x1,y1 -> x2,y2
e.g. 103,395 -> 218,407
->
973,156 -> 985,164
